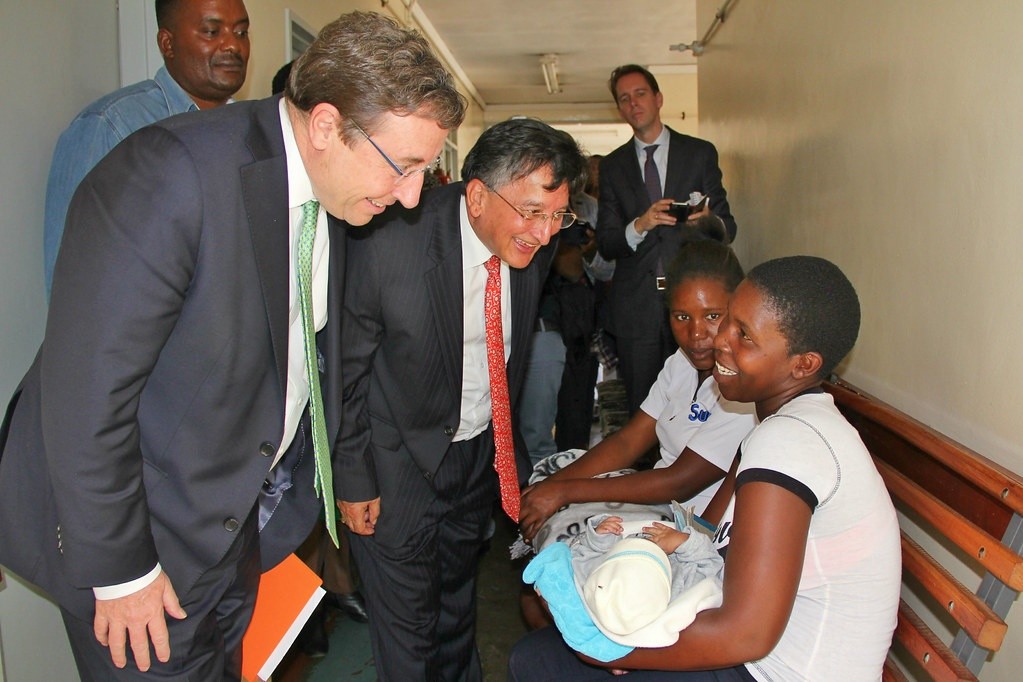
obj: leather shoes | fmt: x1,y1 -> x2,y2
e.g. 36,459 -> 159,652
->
323,590 -> 369,623
293,613 -> 330,656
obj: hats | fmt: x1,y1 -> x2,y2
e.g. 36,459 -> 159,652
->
583,538 -> 672,636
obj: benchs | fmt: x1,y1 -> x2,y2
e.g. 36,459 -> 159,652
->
827,378 -> 1023,682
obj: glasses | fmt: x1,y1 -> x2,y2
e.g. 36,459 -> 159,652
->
339,111 -> 442,185
480,180 -> 577,229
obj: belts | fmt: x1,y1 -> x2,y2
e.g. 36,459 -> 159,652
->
533,317 -> 557,332
655,275 -> 668,290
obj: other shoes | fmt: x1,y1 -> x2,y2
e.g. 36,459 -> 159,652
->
518,593 -> 549,629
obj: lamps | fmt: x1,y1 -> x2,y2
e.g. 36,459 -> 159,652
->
538,53 -> 560,96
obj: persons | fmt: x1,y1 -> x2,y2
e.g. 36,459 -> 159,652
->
0,10 -> 464,682
508,65 -> 903,681
44,0 -> 250,310
334,114 -> 588,680
298,525 -> 369,659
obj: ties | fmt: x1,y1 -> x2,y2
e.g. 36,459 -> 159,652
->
482,255 -> 520,525
297,202 -> 340,549
644,144 -> 664,207
